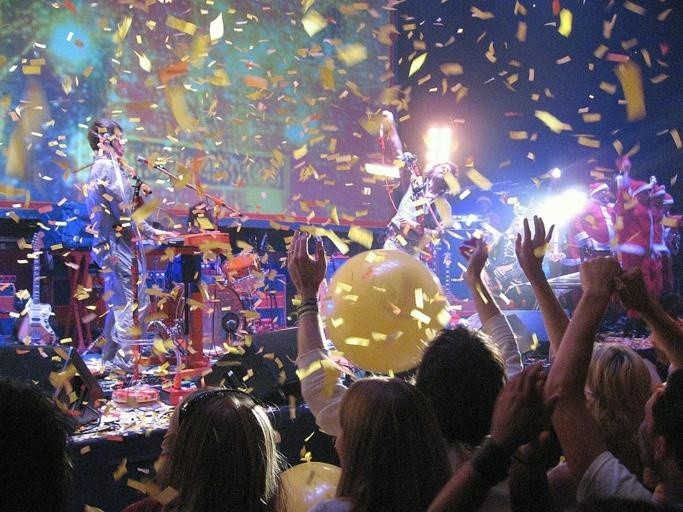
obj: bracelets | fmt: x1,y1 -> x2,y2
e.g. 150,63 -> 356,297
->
470,435 -> 507,488
295,300 -> 318,319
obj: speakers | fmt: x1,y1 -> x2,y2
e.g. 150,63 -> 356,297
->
197,326 -> 302,402
469,308 -> 570,357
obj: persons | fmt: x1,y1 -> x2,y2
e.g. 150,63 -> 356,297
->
652,182 -> 663,300
382,108 -> 459,259
616,157 -> 653,296
288,229 -> 524,460
474,196 -> 501,232
88,117 -> 151,383
426,341 -> 652,512
310,377 -> 450,512
544,255 -> 683,512
0,378 -> 100,511
122,386 -> 276,512
570,182 -> 616,261
664,190 -> 673,293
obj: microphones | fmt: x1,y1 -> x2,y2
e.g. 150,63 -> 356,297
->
102,133 -> 115,159
137,155 -> 165,171
401,151 -> 424,193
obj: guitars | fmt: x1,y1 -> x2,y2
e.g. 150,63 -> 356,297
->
17,232 -> 56,347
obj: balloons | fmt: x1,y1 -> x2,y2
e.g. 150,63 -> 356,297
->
320,249 -> 448,373
276,462 -> 342,512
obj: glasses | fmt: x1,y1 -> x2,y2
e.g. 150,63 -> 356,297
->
178,387 -> 260,426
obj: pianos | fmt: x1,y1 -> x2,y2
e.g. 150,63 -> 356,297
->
135,228 -> 232,258
